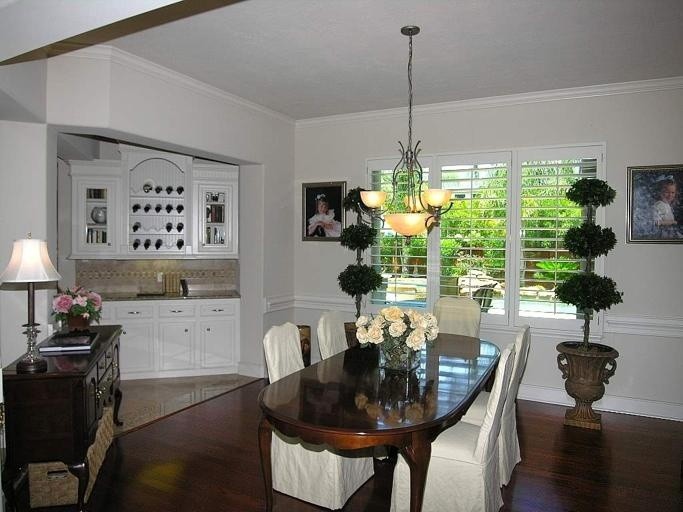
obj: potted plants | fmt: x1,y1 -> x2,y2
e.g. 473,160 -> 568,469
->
556,178 -> 624,431
338,186 -> 383,349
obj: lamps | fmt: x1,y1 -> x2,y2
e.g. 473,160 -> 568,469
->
360,36 -> 454,246
2,233 -> 62,373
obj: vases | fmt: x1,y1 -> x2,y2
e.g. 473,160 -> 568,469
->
68,315 -> 90,331
383,346 -> 421,372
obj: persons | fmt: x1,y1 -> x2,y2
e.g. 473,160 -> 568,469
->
647,179 -> 682,239
307,193 -> 341,238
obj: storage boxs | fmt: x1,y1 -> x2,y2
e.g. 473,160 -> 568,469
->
29,408 -> 114,506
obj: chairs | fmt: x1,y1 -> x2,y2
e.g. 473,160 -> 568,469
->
433,296 -> 481,338
440,285 -> 460,298
473,285 -> 490,306
440,276 -> 458,285
390,344 -> 516,512
464,324 -> 531,486
318,310 -> 351,360
483,283 -> 498,312
263,322 -> 375,510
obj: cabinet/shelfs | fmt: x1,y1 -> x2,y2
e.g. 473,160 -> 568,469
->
119,316 -> 156,380
129,159 -> 188,250
202,304 -> 233,316
197,183 -> 232,250
160,305 -> 194,318
154,316 -> 240,378
117,306 -> 152,317
103,307 -> 109,317
77,179 -> 118,252
3,324 -> 123,511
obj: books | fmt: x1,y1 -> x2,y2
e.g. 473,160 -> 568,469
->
38,331 -> 99,353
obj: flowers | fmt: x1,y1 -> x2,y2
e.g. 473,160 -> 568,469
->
355,306 -> 438,371
51,281 -> 103,325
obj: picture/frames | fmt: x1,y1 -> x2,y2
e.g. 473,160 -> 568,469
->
302,180 -> 346,241
626,165 -> 683,245
